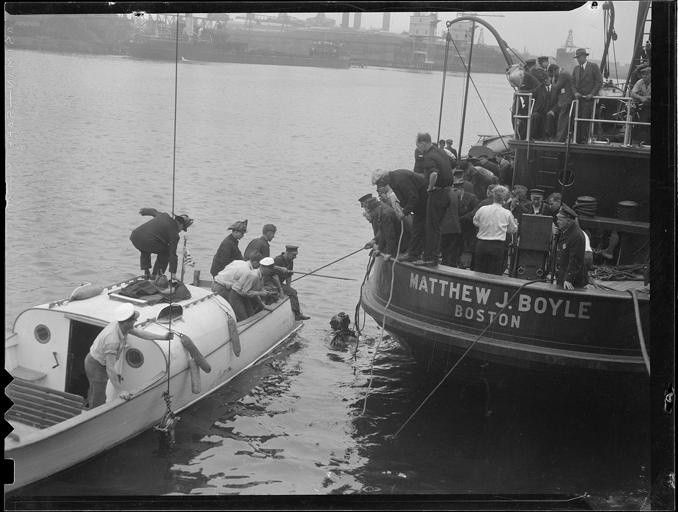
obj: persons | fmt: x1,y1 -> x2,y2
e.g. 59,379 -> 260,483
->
244,224 -> 276,265
211,253 -> 261,300
507,48 -> 602,142
361,131 -> 511,267
473,185 -> 594,291
209,218 -> 249,275
228,255 -> 276,320
82,300 -> 175,411
270,244 -> 310,320
629,67 -> 652,145
128,208 -> 194,278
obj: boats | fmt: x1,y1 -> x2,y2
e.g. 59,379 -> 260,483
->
178,31 -> 352,70
3,271 -> 311,494
356,1 -> 653,377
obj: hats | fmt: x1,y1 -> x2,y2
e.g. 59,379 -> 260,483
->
530,189 -> 545,195
358,169 -> 384,213
285,245 -> 298,255
227,219 -> 248,234
114,303 -> 140,323
573,49 -> 589,58
259,257 -> 275,268
526,56 -> 560,81
175,214 -> 194,232
559,202 -> 578,220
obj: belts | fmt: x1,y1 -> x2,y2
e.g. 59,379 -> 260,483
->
438,187 -> 448,190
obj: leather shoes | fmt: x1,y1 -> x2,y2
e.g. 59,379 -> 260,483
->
412,259 -> 434,267
149,274 -> 161,280
144,273 -> 150,280
295,315 -> 311,321
397,253 -> 420,262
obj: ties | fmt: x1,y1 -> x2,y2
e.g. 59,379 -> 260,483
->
548,87 -> 549,92
582,64 -> 583,69
